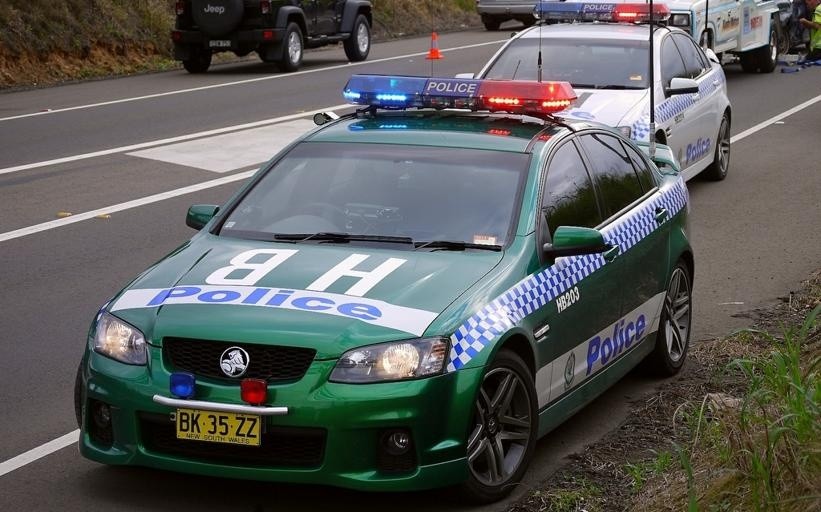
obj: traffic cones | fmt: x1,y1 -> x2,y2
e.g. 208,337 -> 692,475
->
425,32 -> 445,59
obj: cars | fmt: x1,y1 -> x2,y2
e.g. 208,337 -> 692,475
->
455,1 -> 728,182
75,72 -> 694,504
475,0 -> 545,28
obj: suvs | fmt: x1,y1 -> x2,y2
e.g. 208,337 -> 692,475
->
568,0 -> 792,71
169,0 -> 373,71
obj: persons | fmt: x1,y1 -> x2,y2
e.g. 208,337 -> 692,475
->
799,0 -> 821,65
